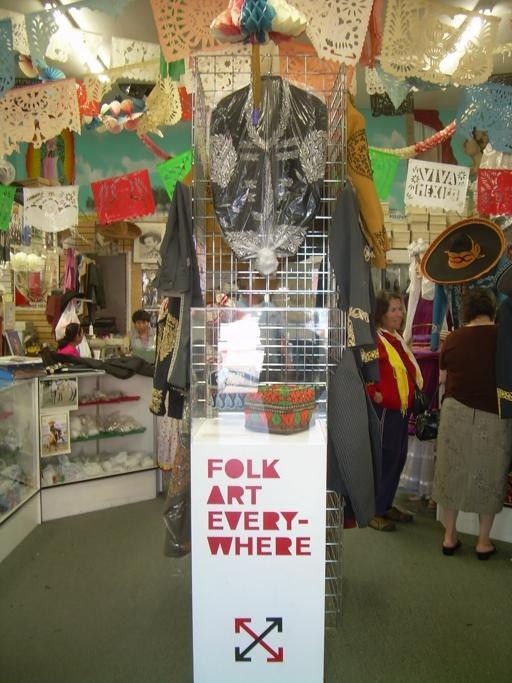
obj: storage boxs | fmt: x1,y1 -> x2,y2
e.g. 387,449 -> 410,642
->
379,203 -> 468,250
31,321 -> 57,344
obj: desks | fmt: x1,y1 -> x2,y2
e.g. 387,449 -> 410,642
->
88,337 -> 130,360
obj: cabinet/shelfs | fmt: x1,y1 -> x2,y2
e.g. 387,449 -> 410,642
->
0,372 -> 159,566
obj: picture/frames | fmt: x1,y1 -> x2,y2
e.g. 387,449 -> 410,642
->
134,220 -> 167,265
4,331 -> 25,357
141,269 -> 163,310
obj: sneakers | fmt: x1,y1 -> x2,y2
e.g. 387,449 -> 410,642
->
386,506 -> 414,524
367,517 -> 396,533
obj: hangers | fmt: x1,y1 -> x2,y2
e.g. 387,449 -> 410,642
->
242,53 -> 289,91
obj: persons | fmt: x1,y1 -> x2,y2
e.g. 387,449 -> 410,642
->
362,290 -> 423,533
127,309 -> 158,359
139,231 -> 162,260
431,285 -> 512,559
56,322 -> 85,358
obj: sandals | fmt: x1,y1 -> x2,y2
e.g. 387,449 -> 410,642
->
475,541 -> 499,560
442,538 -> 462,556
